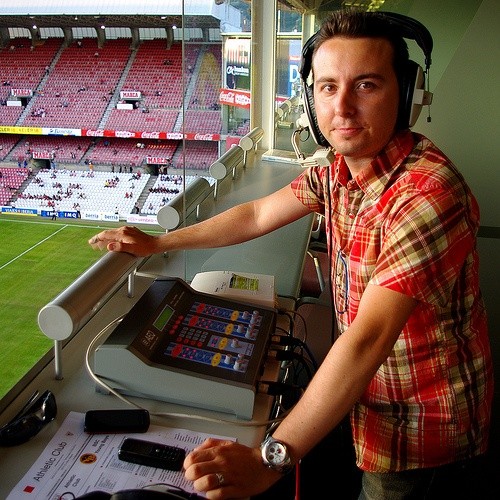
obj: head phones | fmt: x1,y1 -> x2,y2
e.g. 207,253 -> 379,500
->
297,11 -> 434,146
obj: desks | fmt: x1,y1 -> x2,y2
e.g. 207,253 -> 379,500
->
0,145 -> 319,500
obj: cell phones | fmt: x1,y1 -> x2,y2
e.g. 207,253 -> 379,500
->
84,409 -> 151,433
118,437 -> 186,471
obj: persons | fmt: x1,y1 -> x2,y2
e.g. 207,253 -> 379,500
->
88,9 -> 493,500
0,138 -> 182,221
0,33 -> 219,115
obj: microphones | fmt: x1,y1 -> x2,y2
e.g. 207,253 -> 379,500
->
291,127 -> 336,167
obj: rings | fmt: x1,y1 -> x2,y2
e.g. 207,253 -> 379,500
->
92,236 -> 99,242
214,471 -> 226,486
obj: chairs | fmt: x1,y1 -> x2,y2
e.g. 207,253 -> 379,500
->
303,235 -> 332,289
296,292 -> 339,366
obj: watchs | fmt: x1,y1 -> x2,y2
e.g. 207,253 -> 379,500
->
260,436 -> 293,473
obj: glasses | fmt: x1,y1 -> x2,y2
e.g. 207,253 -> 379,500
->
0,389 -> 58,448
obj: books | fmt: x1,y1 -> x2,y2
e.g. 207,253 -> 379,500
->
261,147 -> 308,167
